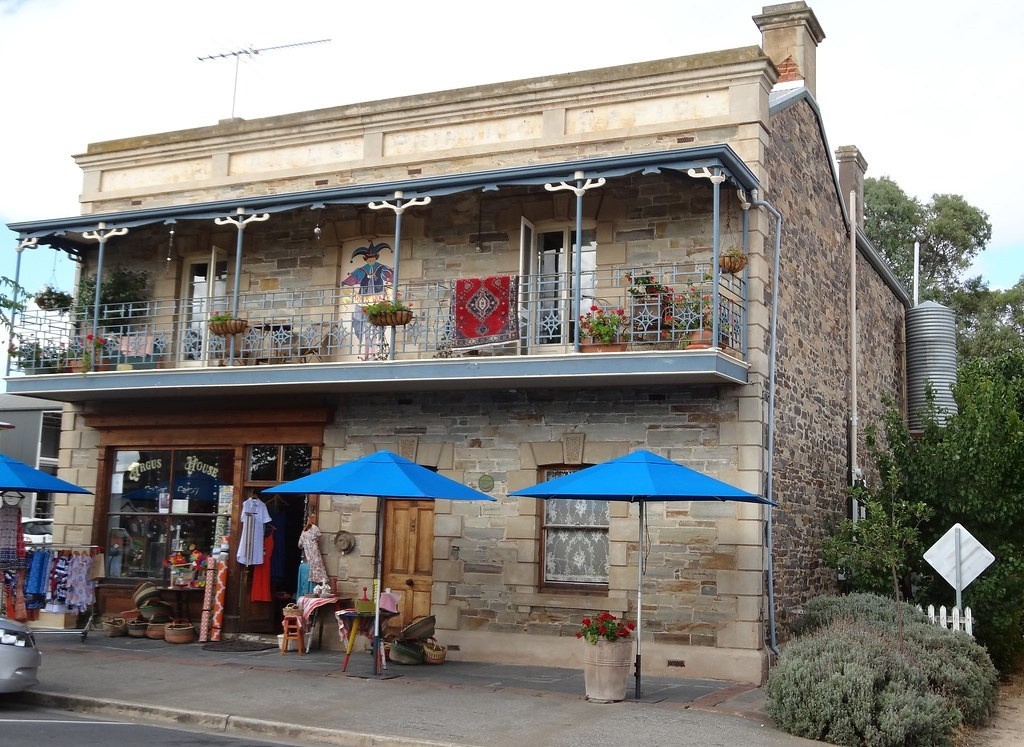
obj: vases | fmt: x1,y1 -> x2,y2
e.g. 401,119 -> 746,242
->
24,360 -> 110,376
579,344 -> 628,353
368,313 -> 412,326
630,300 -> 677,326
685,328 -> 720,349
208,320 -> 248,334
583,635 -> 635,700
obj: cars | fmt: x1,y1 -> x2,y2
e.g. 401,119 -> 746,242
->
0,617 -> 42,693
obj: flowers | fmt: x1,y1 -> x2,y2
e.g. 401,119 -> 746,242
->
208,311 -> 234,321
577,269 -> 735,343
362,292 -> 414,314
574,611 -> 636,644
8,331 -> 107,367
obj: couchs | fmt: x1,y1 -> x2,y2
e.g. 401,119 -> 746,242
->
110,335 -> 163,370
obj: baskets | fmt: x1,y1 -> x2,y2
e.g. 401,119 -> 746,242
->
101,581 -> 195,643
277,632 -> 312,651
389,614 -> 447,664
282,602 -> 304,617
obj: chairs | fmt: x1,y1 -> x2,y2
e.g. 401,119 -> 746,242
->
282,323 -> 338,363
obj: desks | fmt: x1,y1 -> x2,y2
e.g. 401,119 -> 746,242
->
157,586 -> 204,625
248,320 -> 293,364
297,597 -> 400,674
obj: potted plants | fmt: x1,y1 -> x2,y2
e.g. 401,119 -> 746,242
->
34,287 -> 73,311
710,244 -> 748,274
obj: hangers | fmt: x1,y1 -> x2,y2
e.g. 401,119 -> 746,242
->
0,488 -> 25,499
243,489 -> 317,539
29,543 -> 90,557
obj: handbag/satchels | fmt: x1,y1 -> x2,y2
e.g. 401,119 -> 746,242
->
87,547 -> 105,581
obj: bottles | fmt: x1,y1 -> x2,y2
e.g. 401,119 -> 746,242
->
312,584 -> 331,593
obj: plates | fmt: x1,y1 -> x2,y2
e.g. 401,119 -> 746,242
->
304,593 -> 335,597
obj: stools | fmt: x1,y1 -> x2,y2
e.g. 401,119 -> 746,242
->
281,617 -> 305,657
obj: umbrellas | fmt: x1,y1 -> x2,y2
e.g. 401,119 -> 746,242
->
261,449 -> 498,674
504,448 -> 779,698
0,452 -> 95,495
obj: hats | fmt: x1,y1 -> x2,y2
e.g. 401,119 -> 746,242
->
334,530 -> 355,553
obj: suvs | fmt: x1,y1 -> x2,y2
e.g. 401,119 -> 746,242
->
21,516 -> 54,550
